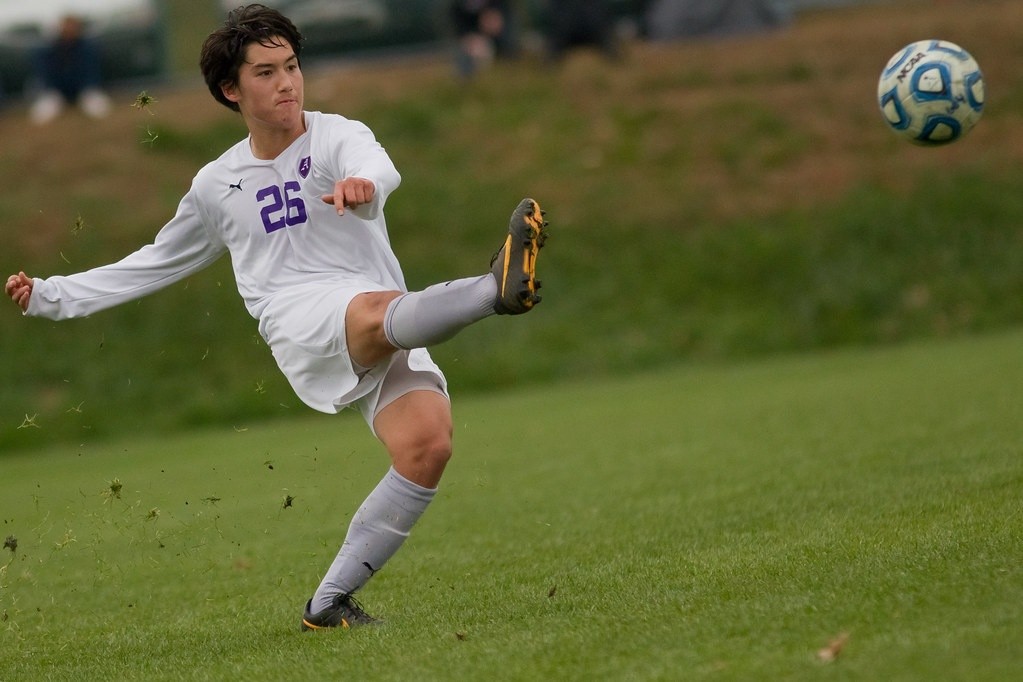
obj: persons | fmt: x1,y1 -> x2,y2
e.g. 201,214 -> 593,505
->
32,16 -> 111,123
452,0 -> 654,66
5,3 -> 549,631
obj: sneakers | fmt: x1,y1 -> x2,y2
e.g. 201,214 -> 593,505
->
489,199 -> 550,316
302,586 -> 381,631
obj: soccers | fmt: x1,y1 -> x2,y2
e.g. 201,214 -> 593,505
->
877,38 -> 986,149
